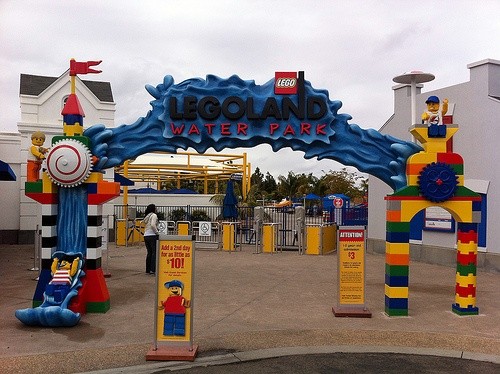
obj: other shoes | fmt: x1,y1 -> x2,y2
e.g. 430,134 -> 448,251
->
146,272 -> 150,275
150,272 -> 155,275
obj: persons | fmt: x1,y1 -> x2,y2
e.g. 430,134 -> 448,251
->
142,203 -> 160,275
